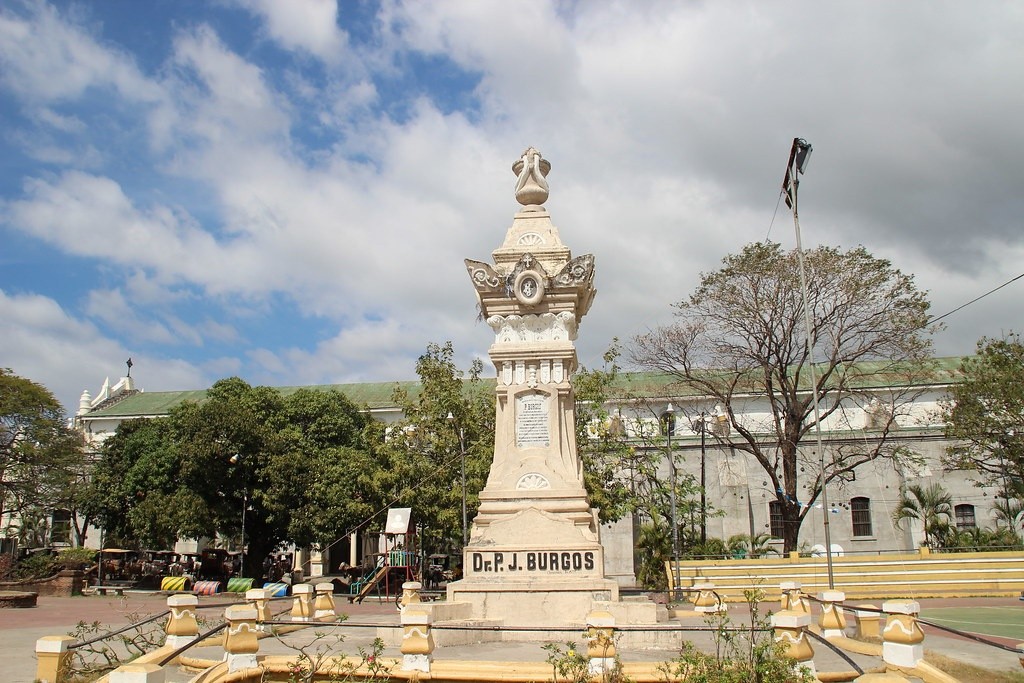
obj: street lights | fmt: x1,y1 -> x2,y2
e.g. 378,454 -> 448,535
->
666,403 -> 681,590
231,454 -> 247,578
782,137 -> 833,591
447,412 -> 467,547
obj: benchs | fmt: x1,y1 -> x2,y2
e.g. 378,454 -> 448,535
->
89,586 -> 129,595
101,580 -> 138,587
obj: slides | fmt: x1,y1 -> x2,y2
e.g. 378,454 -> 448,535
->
347,565 -> 392,604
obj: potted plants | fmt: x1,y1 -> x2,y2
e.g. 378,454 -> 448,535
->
919,539 -> 930,553
60,547 -> 95,571
